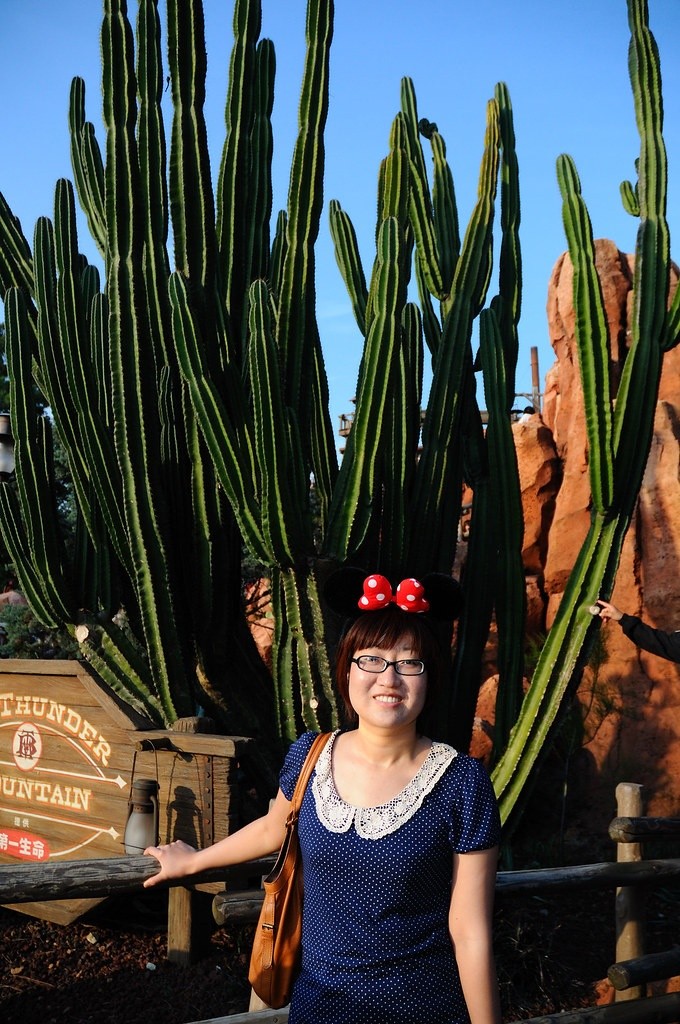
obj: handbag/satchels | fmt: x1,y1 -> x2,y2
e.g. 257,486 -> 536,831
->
247,732 -> 332,1011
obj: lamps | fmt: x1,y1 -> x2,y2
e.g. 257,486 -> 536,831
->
121,737 -> 160,855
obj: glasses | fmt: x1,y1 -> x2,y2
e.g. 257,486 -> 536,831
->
349,655 -> 427,676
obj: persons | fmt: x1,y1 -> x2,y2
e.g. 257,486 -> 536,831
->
142,575 -> 502,1024
